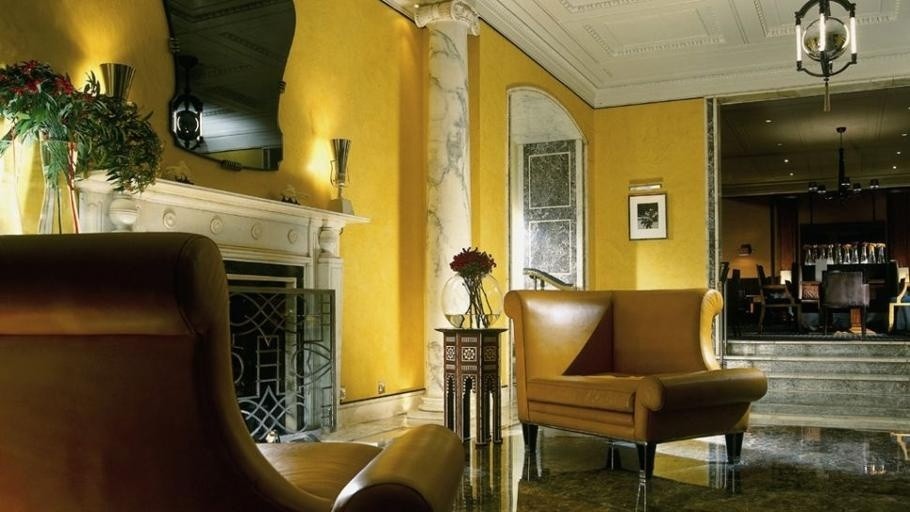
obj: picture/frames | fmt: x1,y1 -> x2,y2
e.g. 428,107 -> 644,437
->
628,192 -> 668,240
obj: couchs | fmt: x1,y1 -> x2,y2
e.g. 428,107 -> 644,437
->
0,232 -> 464,512
503,288 -> 768,481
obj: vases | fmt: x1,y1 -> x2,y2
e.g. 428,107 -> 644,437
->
860,247 -> 868,264
440,272 -> 505,329
37,127 -> 68,233
869,248 -> 877,264
827,251 -> 834,264
819,251 -> 825,259
805,252 -> 812,265
835,249 -> 842,264
843,250 -> 851,264
877,248 -> 886,264
852,249 -> 859,264
813,252 -> 818,265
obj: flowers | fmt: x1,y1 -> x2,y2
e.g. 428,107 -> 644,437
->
803,245 -> 812,262
861,241 -> 869,261
876,243 -> 886,263
449,247 -> 497,328
819,244 -> 826,259
813,245 -> 818,261
828,244 -> 834,258
867,243 -> 877,261
835,243 -> 841,261
852,242 -> 858,261
842,244 -> 851,262
0,60 -> 165,233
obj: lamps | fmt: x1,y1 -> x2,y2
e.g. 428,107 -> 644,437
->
808,127 -> 880,200
794,0 -> 858,113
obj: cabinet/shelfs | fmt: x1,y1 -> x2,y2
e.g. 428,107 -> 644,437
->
802,261 -> 898,314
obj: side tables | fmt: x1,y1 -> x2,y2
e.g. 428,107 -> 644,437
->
434,329 -> 509,445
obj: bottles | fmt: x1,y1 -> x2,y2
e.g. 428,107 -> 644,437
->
805,243 -> 887,264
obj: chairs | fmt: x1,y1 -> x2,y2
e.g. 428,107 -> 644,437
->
885,278 -> 909,333
755,264 -> 804,333
818,270 -> 871,342
720,262 -> 729,281
